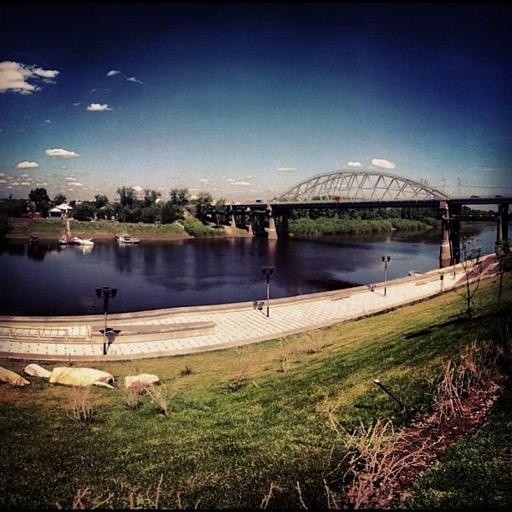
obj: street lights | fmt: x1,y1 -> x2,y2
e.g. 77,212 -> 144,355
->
94,283 -> 118,355
261,264 -> 274,317
382,255 -> 391,298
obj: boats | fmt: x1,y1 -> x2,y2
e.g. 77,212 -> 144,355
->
68,237 -> 95,246
120,233 -> 140,244
59,239 -> 67,244
